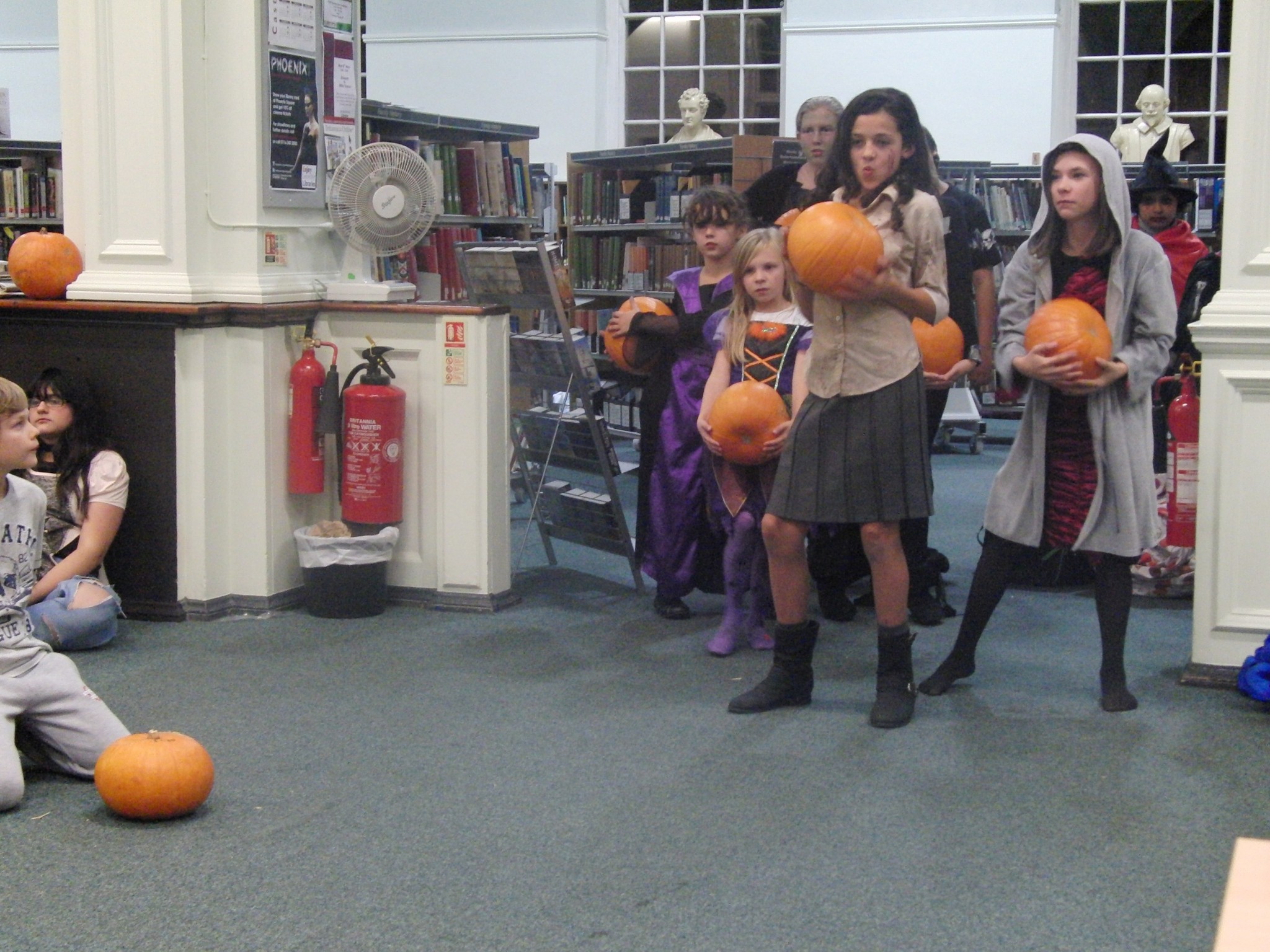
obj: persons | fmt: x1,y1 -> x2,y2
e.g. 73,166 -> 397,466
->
662,86 -> 725,145
726,87 -> 950,726
606,98 -> 1004,658
290,94 -> 319,188
0,363 -> 133,813
1108,84 -> 1196,163
919,133 -> 1177,711
1128,128 -> 1221,474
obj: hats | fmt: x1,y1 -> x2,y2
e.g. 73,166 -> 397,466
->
1129,127 -> 1200,212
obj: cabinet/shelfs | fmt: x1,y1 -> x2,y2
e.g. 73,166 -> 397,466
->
0,139 -> 63,261
933,156 -> 1227,423
360,101 -> 799,598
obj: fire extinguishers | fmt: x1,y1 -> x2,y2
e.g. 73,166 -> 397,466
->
336,347 -> 403,528
1152,353 -> 1201,546
288,311 -> 339,494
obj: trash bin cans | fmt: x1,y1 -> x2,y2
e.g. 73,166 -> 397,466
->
294,522 -> 387,618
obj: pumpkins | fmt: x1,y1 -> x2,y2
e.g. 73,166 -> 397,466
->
93,730 -> 214,821
708,380 -> 790,467
912,317 -> 964,375
604,295 -> 677,375
787,200 -> 884,295
7,227 -> 84,301
775,207 -> 806,227
1024,297 -> 1113,380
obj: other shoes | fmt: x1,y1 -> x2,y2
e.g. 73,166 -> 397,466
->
652,594 -> 695,620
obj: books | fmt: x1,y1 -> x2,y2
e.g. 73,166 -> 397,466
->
356,134 -> 731,550
973,176 -> 1044,232
0,167 -> 62,272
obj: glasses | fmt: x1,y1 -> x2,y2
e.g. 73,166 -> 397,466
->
28,396 -> 72,408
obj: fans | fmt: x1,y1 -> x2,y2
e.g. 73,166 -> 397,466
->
323,140 -> 443,306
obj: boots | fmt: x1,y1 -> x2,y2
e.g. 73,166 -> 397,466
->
728,620 -> 821,712
869,632 -> 917,730
